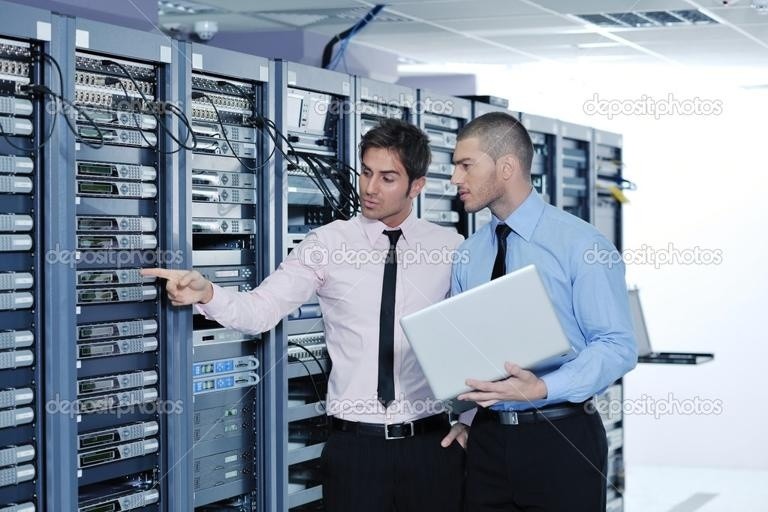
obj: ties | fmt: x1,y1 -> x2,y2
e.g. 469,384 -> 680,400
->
491,224 -> 513,281
377,230 -> 402,411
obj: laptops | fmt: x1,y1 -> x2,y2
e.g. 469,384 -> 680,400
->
399,265 -> 577,402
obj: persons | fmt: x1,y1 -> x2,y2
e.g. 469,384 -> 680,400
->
136,117 -> 478,512
448,109 -> 640,511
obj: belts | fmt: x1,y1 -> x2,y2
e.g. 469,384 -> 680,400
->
498,408 -> 577,428
331,413 -> 444,441
